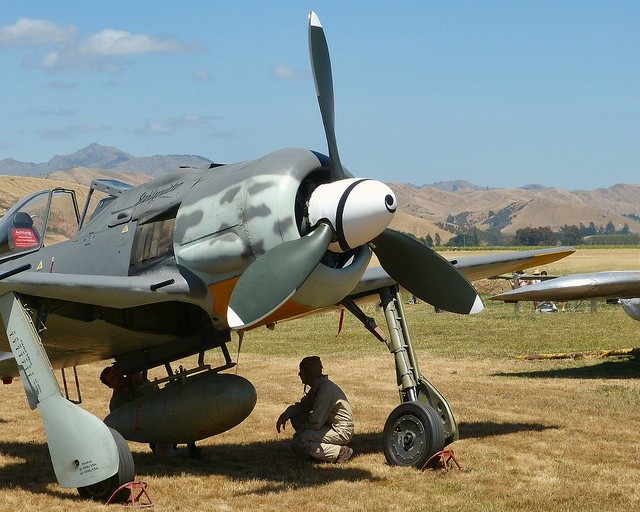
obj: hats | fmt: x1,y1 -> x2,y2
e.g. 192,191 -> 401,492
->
533,269 -> 541,275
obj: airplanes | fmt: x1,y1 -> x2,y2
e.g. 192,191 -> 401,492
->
486,268 -> 640,322
0,11 -> 577,505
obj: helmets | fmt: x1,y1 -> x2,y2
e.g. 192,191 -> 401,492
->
299,356 -> 323,370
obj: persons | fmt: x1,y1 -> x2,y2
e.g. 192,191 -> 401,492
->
515,269 -> 566,312
100,361 -> 204,457
276,356 -> 354,463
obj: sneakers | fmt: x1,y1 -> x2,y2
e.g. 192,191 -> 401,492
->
336,446 -> 354,465
